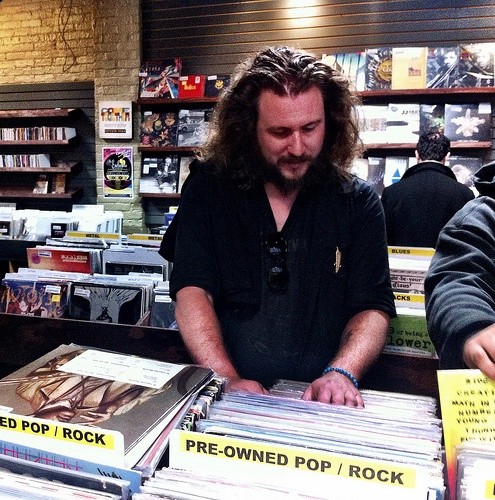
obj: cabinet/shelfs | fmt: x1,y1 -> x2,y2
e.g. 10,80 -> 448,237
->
137,86 -> 495,197
0,109 -> 79,198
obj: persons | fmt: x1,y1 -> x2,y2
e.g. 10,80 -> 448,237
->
429,49 -> 494,86
381,129 -> 475,248
155,157 -> 177,191
15,351 -> 175,426
424,158 -> 495,380
101,108 -> 130,121
156,45 -> 398,409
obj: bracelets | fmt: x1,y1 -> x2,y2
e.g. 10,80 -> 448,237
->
323,366 -> 363,390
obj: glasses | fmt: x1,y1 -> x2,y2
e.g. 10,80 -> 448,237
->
264,228 -> 289,292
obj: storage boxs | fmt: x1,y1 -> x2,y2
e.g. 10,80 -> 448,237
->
179,75 -> 231,98
